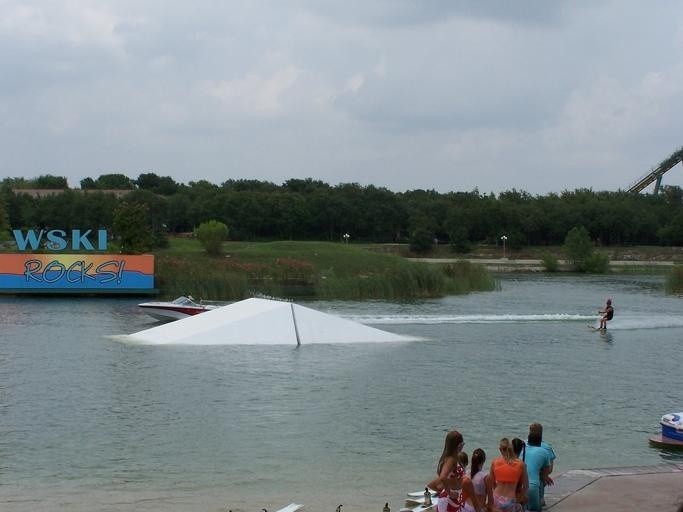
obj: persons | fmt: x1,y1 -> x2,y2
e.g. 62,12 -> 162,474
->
598,298 -> 613,329
427,421 -> 556,512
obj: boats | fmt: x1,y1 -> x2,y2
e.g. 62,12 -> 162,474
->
138,296 -> 220,322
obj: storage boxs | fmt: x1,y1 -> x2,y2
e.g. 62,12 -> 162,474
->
659,412 -> 683,441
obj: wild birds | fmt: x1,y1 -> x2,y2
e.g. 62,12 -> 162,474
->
261,508 -> 267,512
336,505 -> 343,512
383,503 -> 390,512
